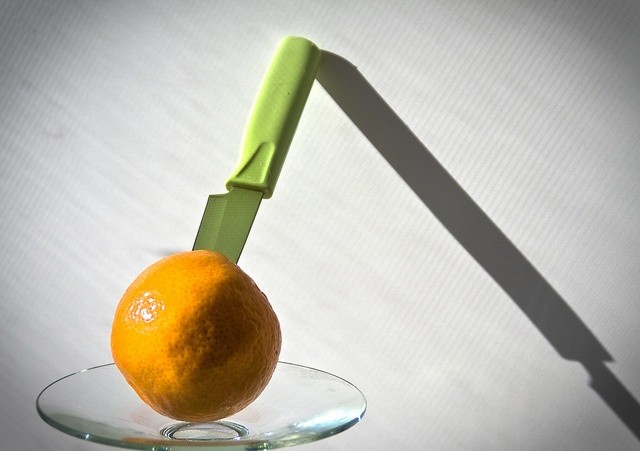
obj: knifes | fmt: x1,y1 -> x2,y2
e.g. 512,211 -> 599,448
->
193,35 -> 321,265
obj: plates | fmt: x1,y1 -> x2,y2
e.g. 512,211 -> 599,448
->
36,357 -> 367,450
